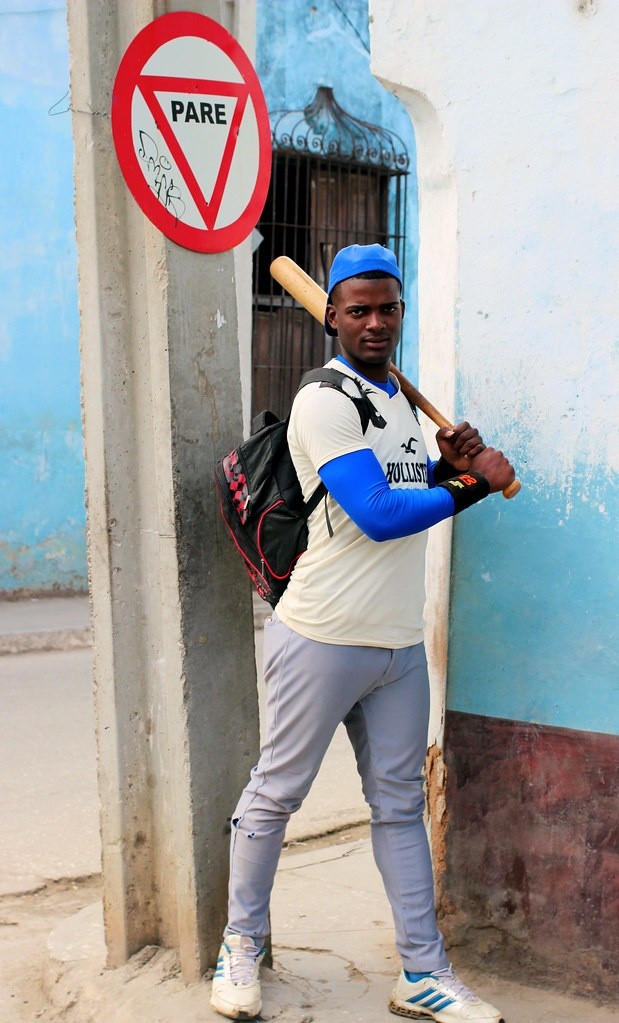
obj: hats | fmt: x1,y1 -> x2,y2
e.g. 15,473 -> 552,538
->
324,244 -> 404,338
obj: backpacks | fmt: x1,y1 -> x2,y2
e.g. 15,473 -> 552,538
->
213,367 -> 386,609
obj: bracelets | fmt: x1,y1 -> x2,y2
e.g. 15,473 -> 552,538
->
437,467 -> 491,517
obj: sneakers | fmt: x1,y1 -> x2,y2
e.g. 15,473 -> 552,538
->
389,965 -> 504,1023
211,934 -> 266,1020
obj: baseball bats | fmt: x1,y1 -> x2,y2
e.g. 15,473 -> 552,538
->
268,254 -> 522,500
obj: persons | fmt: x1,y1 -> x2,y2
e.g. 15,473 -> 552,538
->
211,242 -> 508,1023
